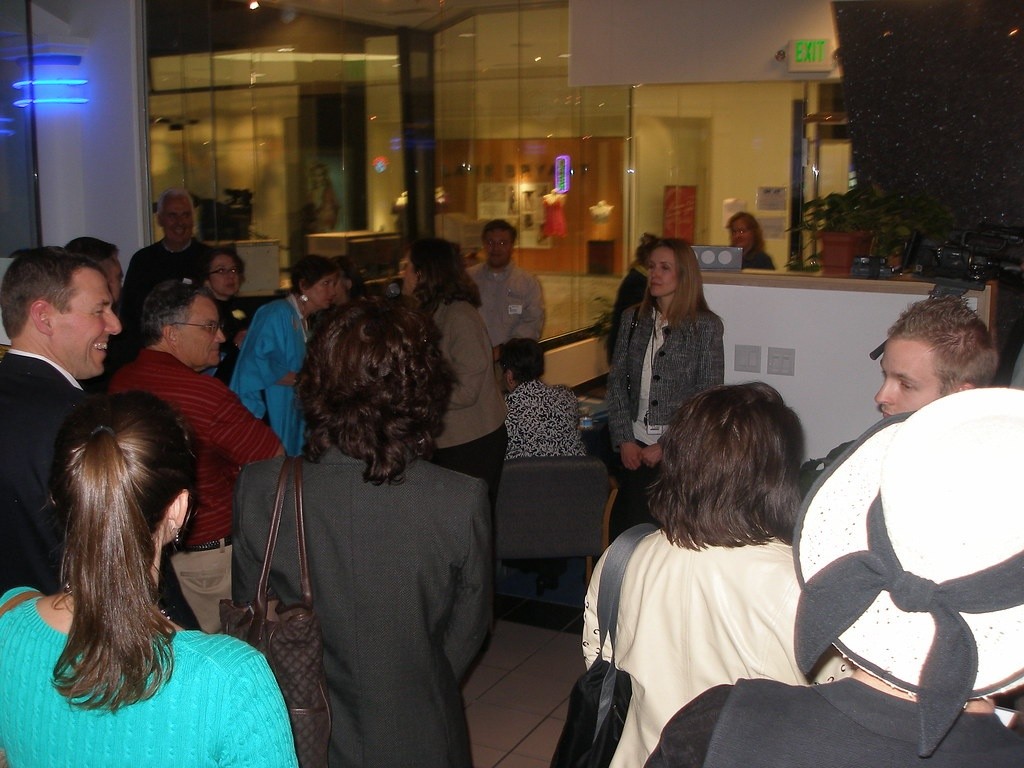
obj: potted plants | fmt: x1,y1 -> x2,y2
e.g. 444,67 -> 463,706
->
786,184 -> 884,274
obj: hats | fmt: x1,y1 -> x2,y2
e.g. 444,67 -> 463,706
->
791,388 -> 1024,756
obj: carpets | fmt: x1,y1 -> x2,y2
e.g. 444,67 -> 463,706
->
496,392 -> 640,636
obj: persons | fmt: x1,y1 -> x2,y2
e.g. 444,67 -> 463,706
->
542,189 -> 567,238
0,188 -> 366,635
403,219 -> 724,546
231,292 -> 494,768
825,297 -> 998,497
645,387 -> 1024,768
582,381 -> 807,768
0,389 -> 299,768
306,159 -> 337,231
726,212 -> 776,270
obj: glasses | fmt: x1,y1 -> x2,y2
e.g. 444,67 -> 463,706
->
208,268 -> 240,276
173,321 -> 223,335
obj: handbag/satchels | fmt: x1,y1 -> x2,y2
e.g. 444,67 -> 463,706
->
219,456 -> 332,768
546,660 -> 633,768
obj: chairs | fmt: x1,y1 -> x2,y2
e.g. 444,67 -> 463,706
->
495,457 -> 618,588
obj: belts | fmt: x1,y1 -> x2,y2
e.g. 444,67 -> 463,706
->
174,532 -> 235,554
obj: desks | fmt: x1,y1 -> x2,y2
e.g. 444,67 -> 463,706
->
307,230 -> 402,285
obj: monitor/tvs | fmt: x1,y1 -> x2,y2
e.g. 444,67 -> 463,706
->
690,246 -> 744,270
